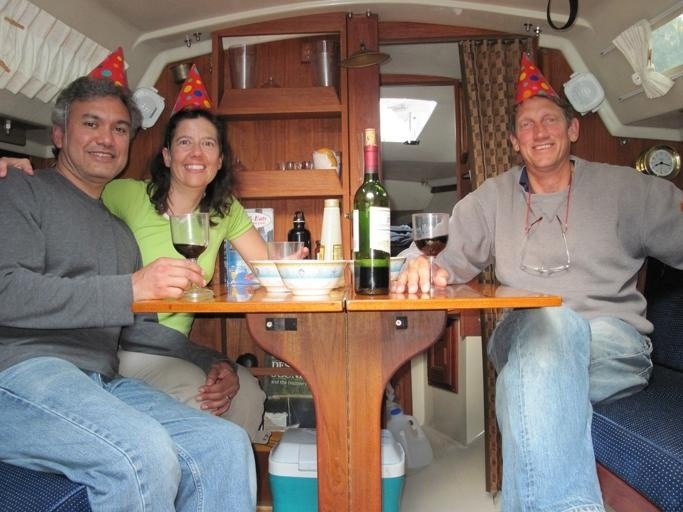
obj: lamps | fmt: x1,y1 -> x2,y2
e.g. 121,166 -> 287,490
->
132,88 -> 165,128
562,72 -> 604,113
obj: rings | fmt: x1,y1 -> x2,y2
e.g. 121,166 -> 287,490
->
226,395 -> 231,400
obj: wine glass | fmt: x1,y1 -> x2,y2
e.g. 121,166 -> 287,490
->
168,213 -> 215,299
412,212 -> 452,293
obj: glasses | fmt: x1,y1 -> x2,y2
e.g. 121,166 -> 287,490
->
519,215 -> 570,278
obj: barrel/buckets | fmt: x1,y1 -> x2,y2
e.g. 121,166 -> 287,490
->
386,408 -> 434,469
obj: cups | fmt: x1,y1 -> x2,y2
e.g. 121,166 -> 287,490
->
229,44 -> 256,90
310,38 -> 338,87
171,63 -> 193,84
267,242 -> 305,258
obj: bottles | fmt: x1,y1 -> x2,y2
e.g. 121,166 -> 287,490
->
288,128 -> 392,295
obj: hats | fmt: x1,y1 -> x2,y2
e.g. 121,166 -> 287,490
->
174,61 -> 221,114
85,42 -> 131,90
515,52 -> 558,101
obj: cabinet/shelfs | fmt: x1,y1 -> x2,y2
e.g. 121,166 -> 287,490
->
209,12 -> 348,287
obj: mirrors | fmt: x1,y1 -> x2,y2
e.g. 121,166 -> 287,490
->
547,0 -> 577,30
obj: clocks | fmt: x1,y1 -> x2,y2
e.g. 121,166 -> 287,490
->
632,143 -> 682,181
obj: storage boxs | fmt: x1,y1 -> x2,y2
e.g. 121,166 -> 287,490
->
267,428 -> 407,511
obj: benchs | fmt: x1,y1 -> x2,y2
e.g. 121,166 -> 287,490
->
591,294 -> 682,512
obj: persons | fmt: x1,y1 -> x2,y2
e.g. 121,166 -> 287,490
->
1,107 -> 309,443
3,78 -> 257,511
389,94 -> 683,512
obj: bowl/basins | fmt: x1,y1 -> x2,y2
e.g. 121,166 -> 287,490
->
249,258 -> 408,295
